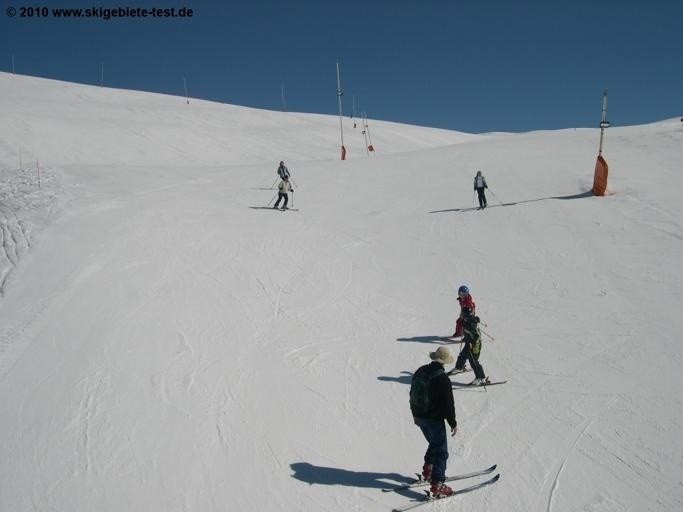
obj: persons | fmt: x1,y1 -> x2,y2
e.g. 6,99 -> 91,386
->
273,174 -> 294,210
277,160 -> 294,192
409,346 -> 460,498
448,306 -> 486,386
473,169 -> 488,210
451,285 -> 476,339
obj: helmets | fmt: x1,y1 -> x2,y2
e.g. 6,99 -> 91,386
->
461,309 -> 473,318
458,287 -> 469,297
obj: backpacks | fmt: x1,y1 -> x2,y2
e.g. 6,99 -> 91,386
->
409,369 -> 444,414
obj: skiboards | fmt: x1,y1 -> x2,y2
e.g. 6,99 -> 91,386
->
382,464 -> 500,512
400,367 -> 508,390
278,209 -> 299,211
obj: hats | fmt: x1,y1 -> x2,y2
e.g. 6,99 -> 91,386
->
430,347 -> 454,363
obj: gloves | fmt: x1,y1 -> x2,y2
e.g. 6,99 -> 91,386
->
472,343 -> 480,355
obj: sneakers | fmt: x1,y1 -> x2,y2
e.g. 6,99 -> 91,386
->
430,481 -> 453,496
449,367 -> 464,376
472,378 -> 486,386
423,464 -> 432,481
